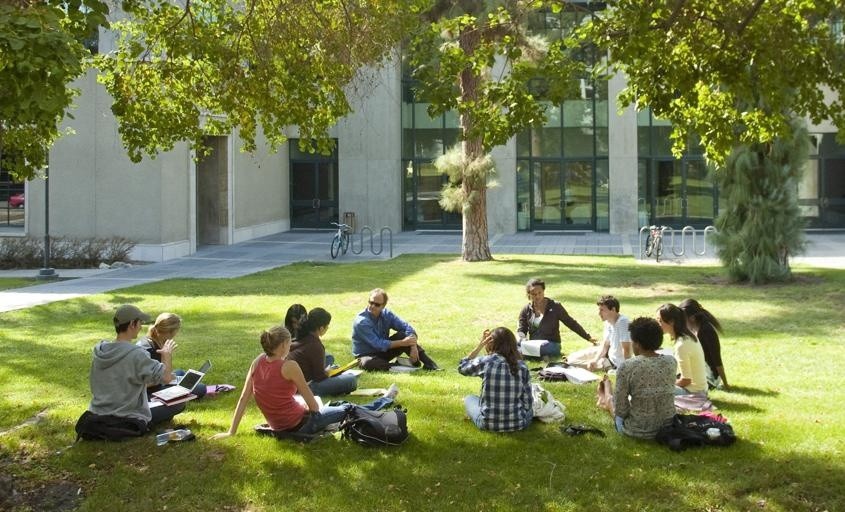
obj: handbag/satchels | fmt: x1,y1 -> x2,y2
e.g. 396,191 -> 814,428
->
530,381 -> 567,425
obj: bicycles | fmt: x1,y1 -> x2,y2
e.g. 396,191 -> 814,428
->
326,220 -> 353,260
644,223 -> 671,262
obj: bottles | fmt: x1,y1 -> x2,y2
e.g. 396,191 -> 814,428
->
156,429 -> 193,447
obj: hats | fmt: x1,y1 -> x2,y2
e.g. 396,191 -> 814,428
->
112,303 -> 152,327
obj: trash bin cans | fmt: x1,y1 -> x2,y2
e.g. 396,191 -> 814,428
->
343,211 -> 356,235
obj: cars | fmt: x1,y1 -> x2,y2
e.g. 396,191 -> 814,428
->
10,191 -> 24,209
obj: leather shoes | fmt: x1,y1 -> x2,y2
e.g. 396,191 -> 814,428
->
423,362 -> 446,371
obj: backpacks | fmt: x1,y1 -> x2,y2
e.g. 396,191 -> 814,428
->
672,413 -> 737,447
338,404 -> 409,449
538,361 -> 570,382
74,409 -> 149,443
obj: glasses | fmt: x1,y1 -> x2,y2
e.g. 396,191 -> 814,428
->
368,297 -> 384,308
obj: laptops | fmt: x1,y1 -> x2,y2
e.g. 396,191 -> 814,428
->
167,359 -> 212,385
151,369 -> 205,402
